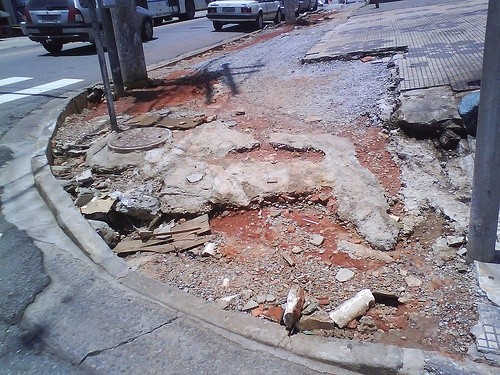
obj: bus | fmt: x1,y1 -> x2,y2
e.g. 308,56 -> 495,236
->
141,0 -> 208,27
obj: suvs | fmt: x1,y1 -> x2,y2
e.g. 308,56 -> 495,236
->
21,1 -> 152,50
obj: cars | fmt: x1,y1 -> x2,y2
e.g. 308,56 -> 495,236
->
207,0 -> 281,29
289,0 -> 318,16
